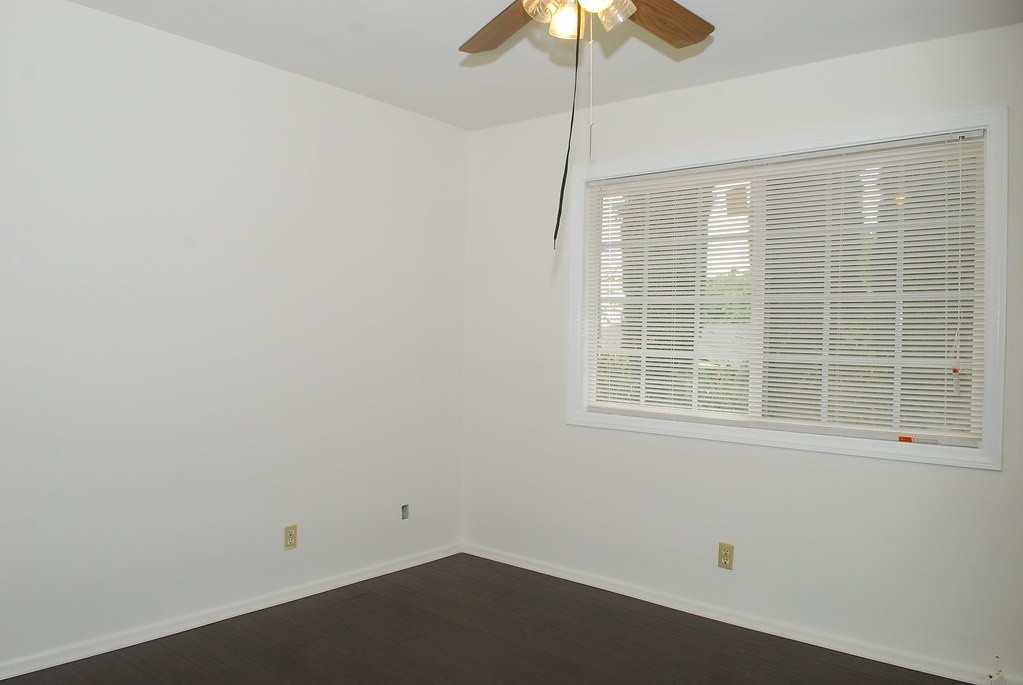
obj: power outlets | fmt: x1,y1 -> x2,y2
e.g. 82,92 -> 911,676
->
284,524 -> 297,550
718,541 -> 734,570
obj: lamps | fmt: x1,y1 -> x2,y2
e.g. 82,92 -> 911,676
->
457,0 -> 714,251
